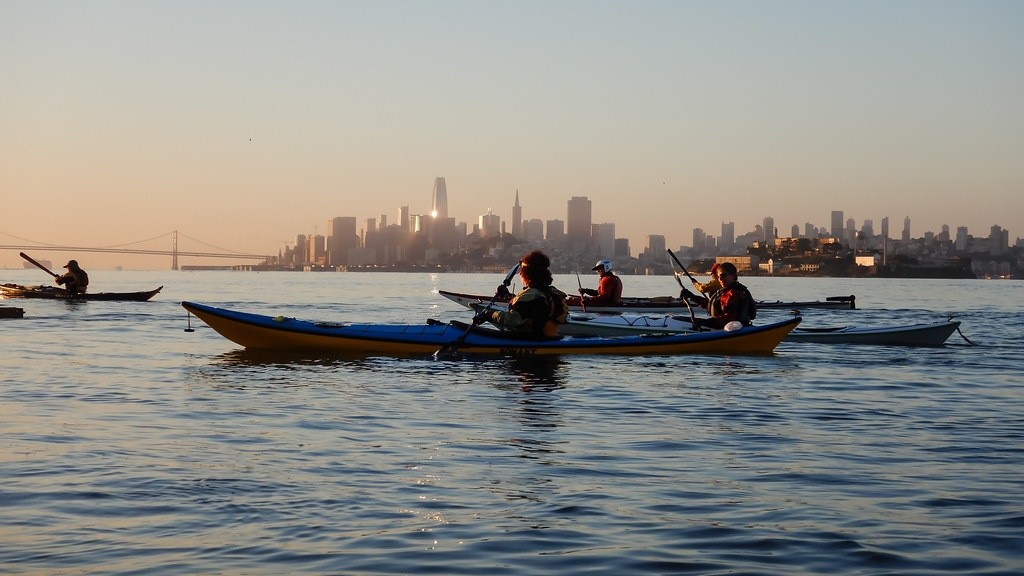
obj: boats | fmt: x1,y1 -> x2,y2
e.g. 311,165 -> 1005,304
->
468,298 -> 963,349
0,280 -> 163,303
438,290 -> 857,315
181,279 -> 802,363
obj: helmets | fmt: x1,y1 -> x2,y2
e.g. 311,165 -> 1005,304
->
592,259 -> 612,272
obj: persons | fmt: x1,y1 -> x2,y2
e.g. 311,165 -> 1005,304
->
491,251 -> 570,340
566,260 -> 623,307
692,263 -> 723,297
55,259 -> 89,294
698,261 -> 758,331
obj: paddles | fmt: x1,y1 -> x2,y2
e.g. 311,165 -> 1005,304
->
575,270 -> 588,313
432,263 -> 522,361
668,248 -> 711,334
20,252 -> 58,278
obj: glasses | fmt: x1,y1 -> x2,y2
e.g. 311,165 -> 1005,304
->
716,273 -> 727,282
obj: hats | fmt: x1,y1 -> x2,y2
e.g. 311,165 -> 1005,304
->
63,260 -> 78,268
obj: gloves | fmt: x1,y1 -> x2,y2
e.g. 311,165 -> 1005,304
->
692,318 -> 705,330
680,289 -> 692,300
496,285 -> 516,302
472,310 -> 496,325
